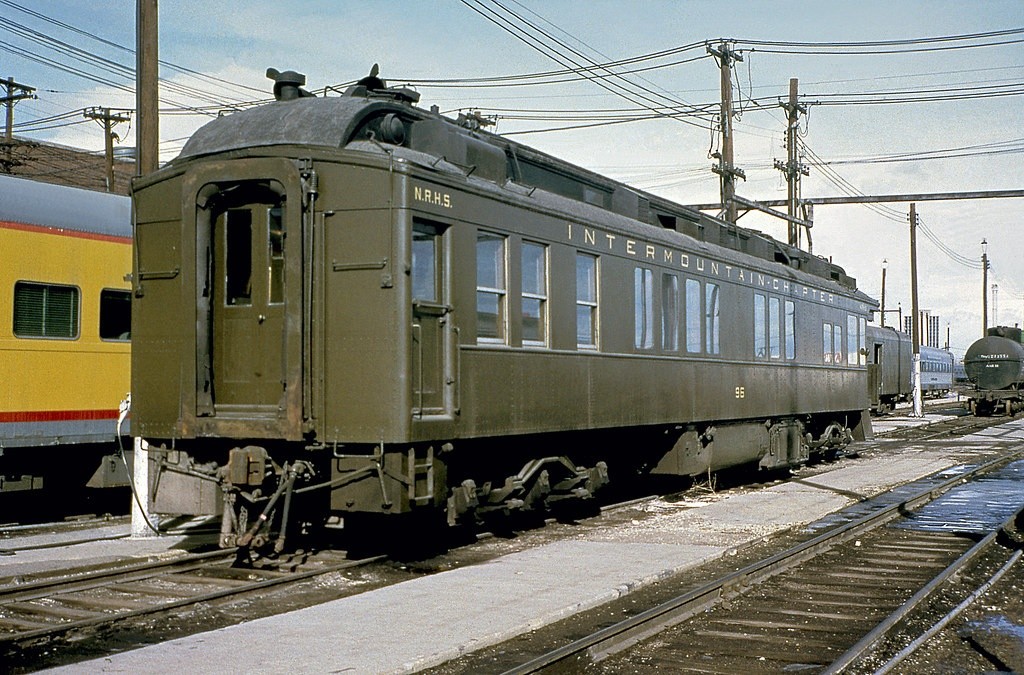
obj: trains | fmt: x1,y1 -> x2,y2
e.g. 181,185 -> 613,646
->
122,63 -> 882,553
0,173 -> 968,484
956,323 -> 1024,416
